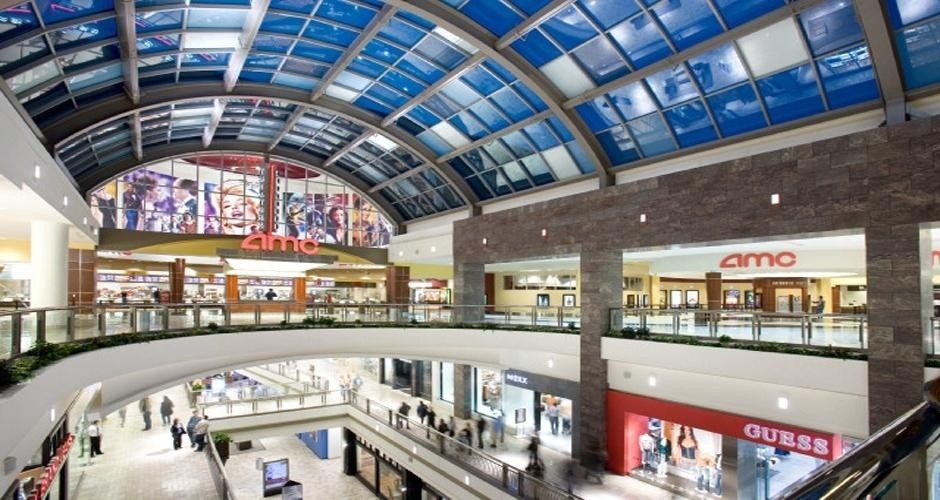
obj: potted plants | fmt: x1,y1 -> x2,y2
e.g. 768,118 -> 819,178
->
213,429 -> 234,464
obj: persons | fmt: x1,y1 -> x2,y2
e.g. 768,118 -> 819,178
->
90,172 -> 391,248
812,296 -> 825,319
90,420 -> 104,458
171,411 -> 210,452
119,408 -> 126,427
154,288 -> 160,304
639,424 -> 722,498
286,360 -> 362,399
398,401 -> 502,461
121,291 -> 128,302
139,397 -> 152,431
77,422 -> 88,458
265,288 -> 277,301
525,394 -> 608,485
161,396 -> 174,426
